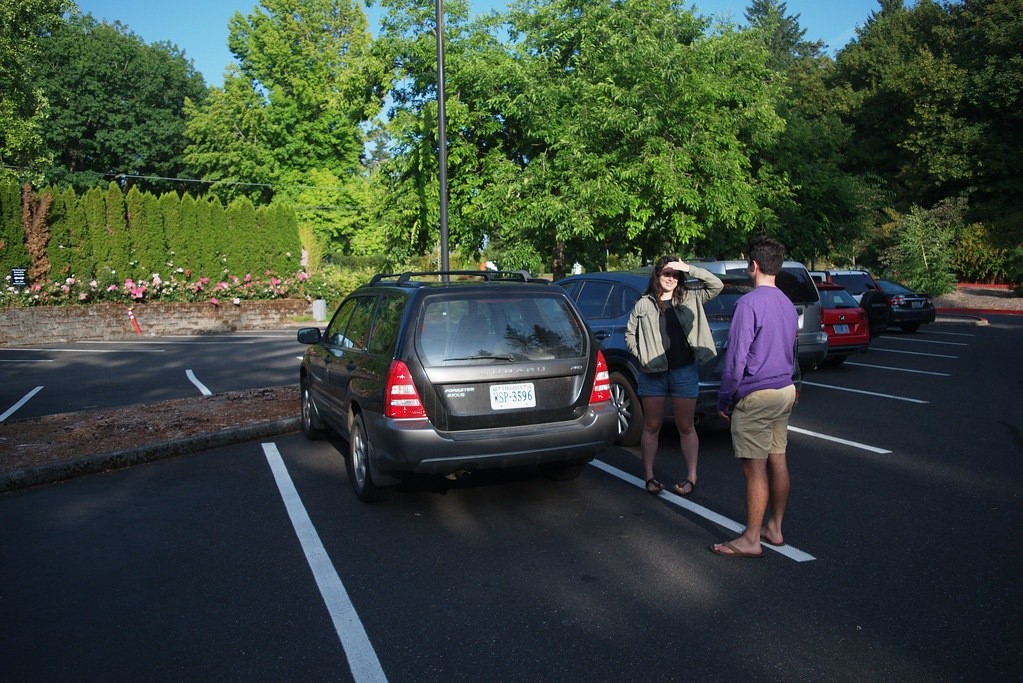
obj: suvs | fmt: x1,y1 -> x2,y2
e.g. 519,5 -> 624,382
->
296,269 -> 619,504
633,258 -> 830,373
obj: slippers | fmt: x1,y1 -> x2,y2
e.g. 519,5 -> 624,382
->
710,540 -> 764,557
741,530 -> 784,546
675,478 -> 693,494
646,477 -> 664,492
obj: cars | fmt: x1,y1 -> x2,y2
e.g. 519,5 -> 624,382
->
808,269 -> 936,357
506,270 -> 801,448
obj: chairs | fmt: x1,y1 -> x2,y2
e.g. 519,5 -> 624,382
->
455,313 -> 489,335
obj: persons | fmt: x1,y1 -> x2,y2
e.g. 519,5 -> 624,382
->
300,245 -> 308,274
625,255 -> 724,495
571,259 -> 584,276
709,235 -> 798,557
